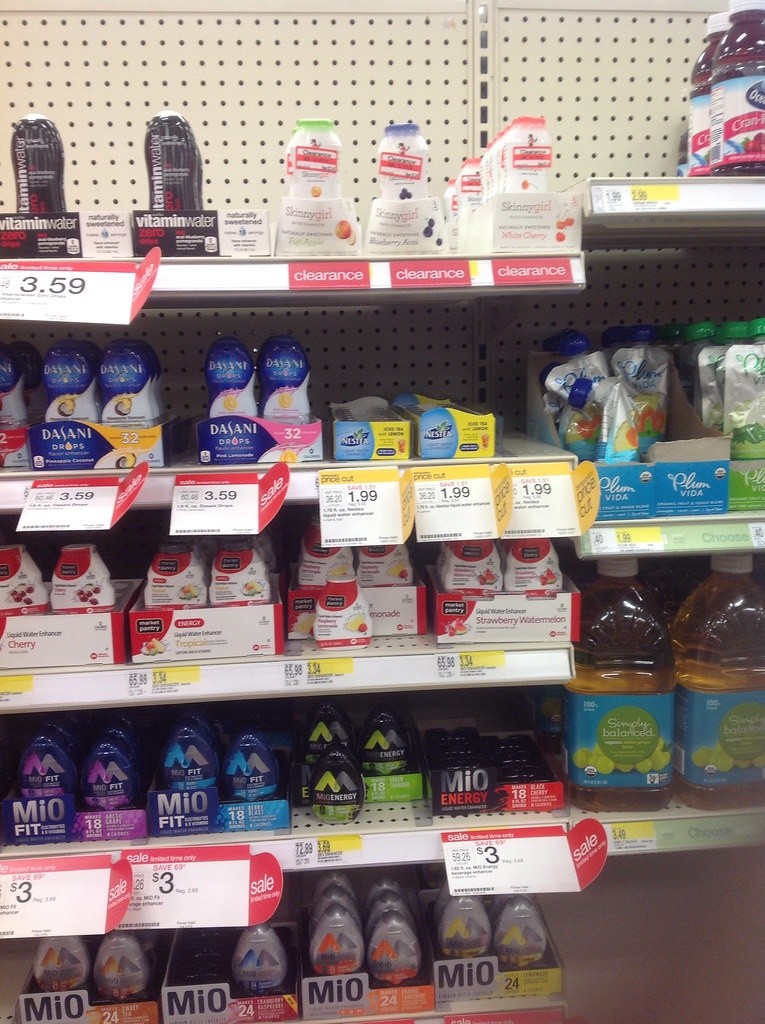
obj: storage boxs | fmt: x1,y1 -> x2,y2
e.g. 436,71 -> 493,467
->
527,351 -> 765,521
292,725 -> 563,815
16,863 -> 564,1024
1,194 -> 584,259
197,414 -> 323,465
0,413 -> 181,469
0,562 -> 581,669
0,733 -> 292,844
328,394 -> 496,460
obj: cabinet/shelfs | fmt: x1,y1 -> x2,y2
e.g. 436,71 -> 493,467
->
0,0 -> 587,1024
475,0 -> 765,855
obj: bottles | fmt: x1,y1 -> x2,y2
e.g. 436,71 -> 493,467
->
560,553 -> 765,812
687,0 -> 765,177
284,116 -> 552,201
